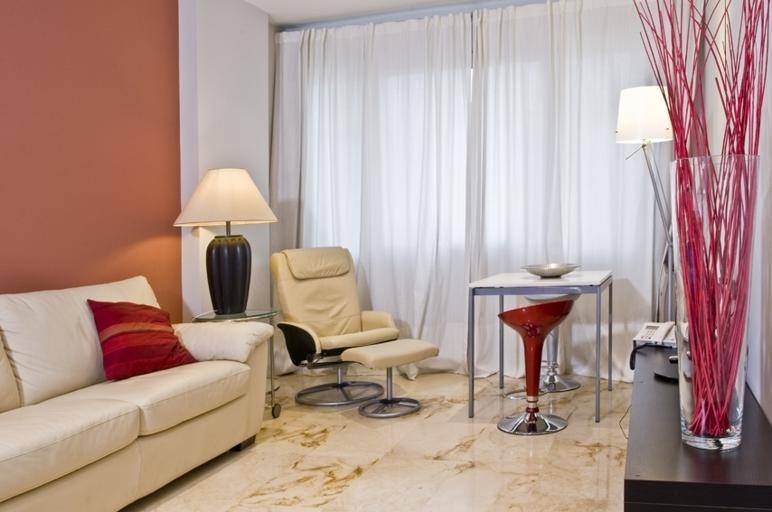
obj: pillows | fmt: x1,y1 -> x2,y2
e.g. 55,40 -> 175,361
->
171,322 -> 275,363
87,299 -> 197,381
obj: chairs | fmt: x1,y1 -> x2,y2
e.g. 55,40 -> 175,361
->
269,246 -> 399,407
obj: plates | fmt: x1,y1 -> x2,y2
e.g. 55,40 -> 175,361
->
521,262 -> 579,279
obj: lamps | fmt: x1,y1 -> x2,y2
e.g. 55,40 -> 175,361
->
615,87 -> 673,322
173,168 -> 278,315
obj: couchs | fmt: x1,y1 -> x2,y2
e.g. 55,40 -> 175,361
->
0,275 -> 274,512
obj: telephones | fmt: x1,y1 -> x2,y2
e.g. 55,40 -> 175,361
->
632,321 -> 678,349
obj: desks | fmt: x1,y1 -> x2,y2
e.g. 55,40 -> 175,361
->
468,271 -> 612,421
191,309 -> 280,418
625,345 -> 771,509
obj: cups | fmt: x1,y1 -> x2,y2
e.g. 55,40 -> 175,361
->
669,155 -> 758,449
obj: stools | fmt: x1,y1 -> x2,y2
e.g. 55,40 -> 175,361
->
523,294 -> 581,392
497,300 -> 575,435
341,339 -> 439,418
